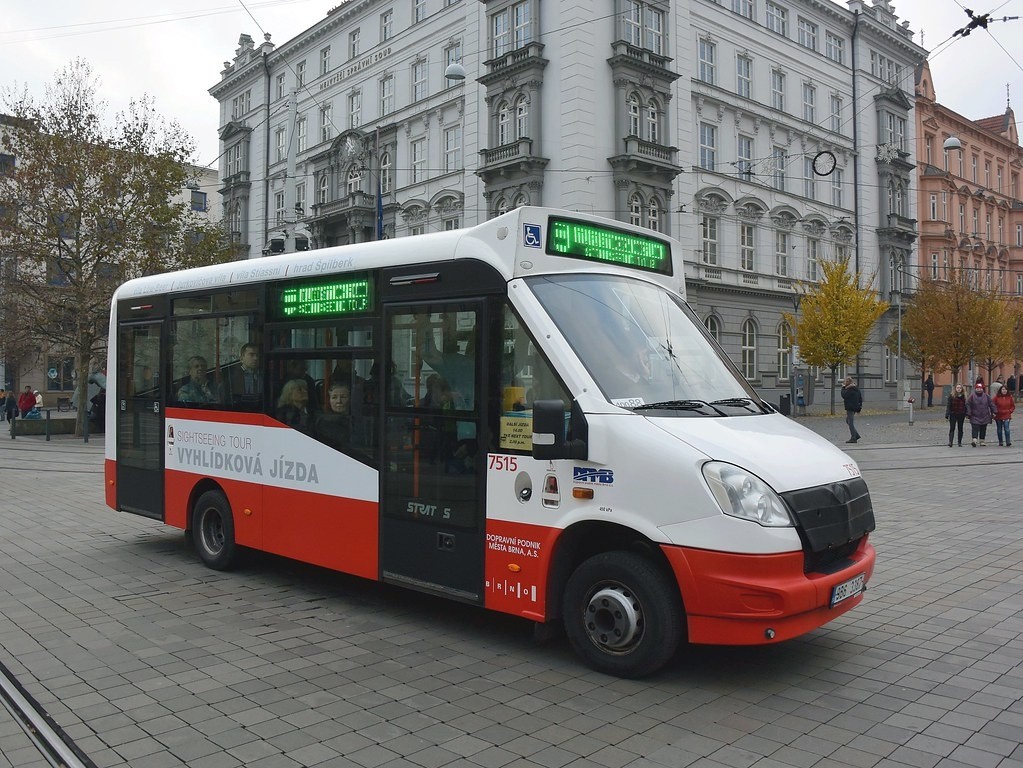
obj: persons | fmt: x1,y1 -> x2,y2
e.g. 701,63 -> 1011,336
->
840,376 -> 863,443
944,383 -> 969,447
975,373 -> 1017,402
989,375 -> 1006,423
922,374 -> 935,407
173,312 -> 736,525
991,385 -> 1016,447
0,385 -> 44,431
965,383 -> 997,447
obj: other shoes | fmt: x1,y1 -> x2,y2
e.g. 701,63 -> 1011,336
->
947,438 -> 1011,448
845,434 -> 862,443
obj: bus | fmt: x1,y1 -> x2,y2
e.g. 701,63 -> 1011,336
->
101,205 -> 880,685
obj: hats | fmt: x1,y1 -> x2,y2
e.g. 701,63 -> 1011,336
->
975,383 -> 984,389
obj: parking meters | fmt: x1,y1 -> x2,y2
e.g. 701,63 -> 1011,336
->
788,366 -> 815,417
